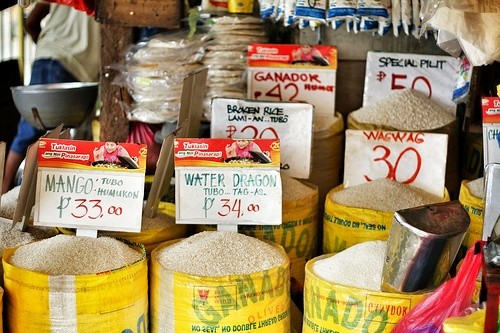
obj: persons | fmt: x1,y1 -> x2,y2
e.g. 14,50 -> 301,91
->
2,0 -> 101,194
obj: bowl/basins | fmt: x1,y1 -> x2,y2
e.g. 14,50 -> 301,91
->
9,80 -> 100,128
